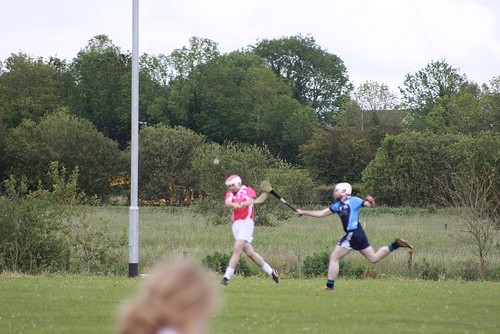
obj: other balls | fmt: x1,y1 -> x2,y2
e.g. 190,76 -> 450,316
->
214,158 -> 219,165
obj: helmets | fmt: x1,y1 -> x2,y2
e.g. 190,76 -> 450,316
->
225,175 -> 241,193
333,182 -> 352,200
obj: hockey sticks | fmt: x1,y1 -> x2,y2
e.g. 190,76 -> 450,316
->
260,179 -> 302,216
231,191 -> 269,210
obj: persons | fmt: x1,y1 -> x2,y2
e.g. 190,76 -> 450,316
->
220,173 -> 281,285
295,182 -> 413,293
118,252 -> 220,334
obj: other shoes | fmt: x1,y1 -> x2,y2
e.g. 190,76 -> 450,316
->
395,237 -> 413,249
221,277 -> 228,286
272,270 -> 279,283
316,285 -> 334,291
